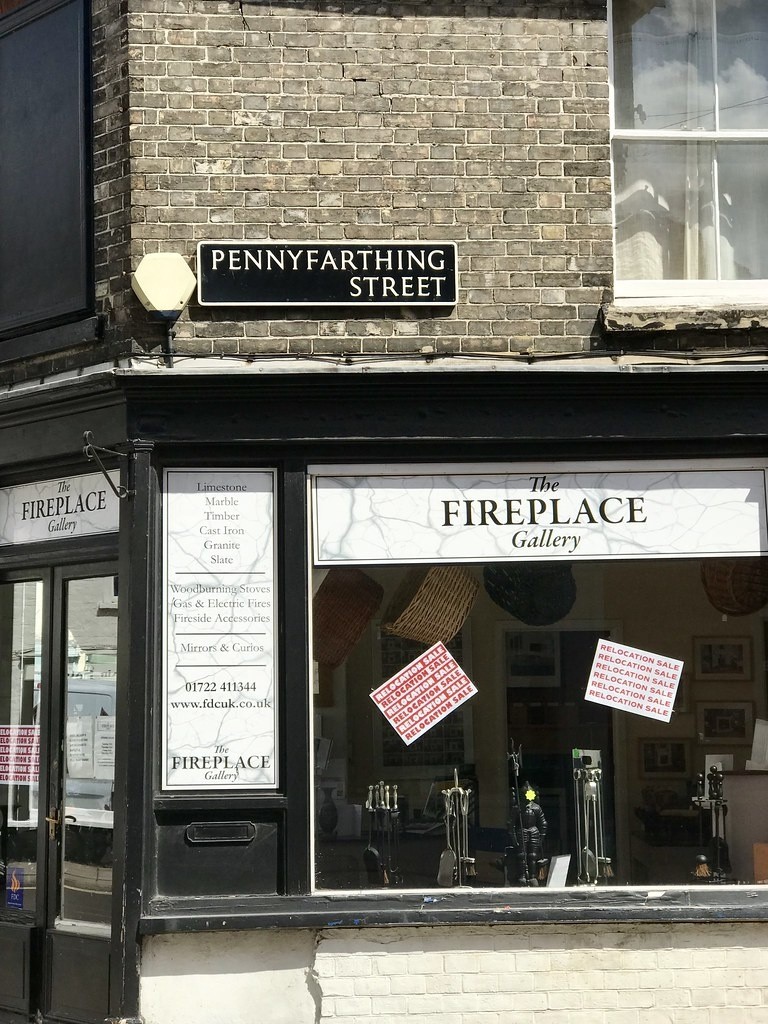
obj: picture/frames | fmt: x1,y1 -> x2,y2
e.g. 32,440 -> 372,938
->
672,674 -> 691,714
695,699 -> 758,747
505,630 -> 560,688
692,634 -> 755,682
637,737 -> 693,782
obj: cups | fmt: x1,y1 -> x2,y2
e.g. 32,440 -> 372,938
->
342,804 -> 362,836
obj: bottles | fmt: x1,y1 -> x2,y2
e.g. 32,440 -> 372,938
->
319,787 -> 337,835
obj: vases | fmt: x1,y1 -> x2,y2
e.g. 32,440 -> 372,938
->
319,786 -> 337,837
